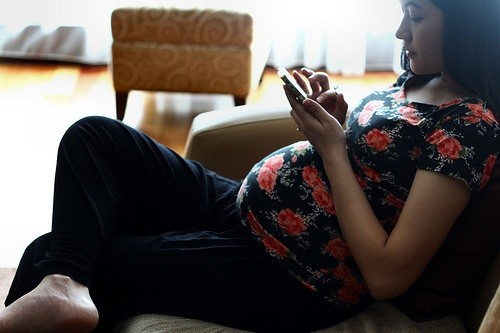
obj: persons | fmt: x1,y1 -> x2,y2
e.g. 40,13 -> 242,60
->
0,0 -> 500,333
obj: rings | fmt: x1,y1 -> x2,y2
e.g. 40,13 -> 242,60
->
331,87 -> 339,95
297,126 -> 300,131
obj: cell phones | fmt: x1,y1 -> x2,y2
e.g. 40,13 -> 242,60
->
278,66 -> 309,104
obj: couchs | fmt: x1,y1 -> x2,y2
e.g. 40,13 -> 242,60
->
120,101 -> 500,333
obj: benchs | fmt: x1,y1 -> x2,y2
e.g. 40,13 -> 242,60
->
110,8 -> 272,121
